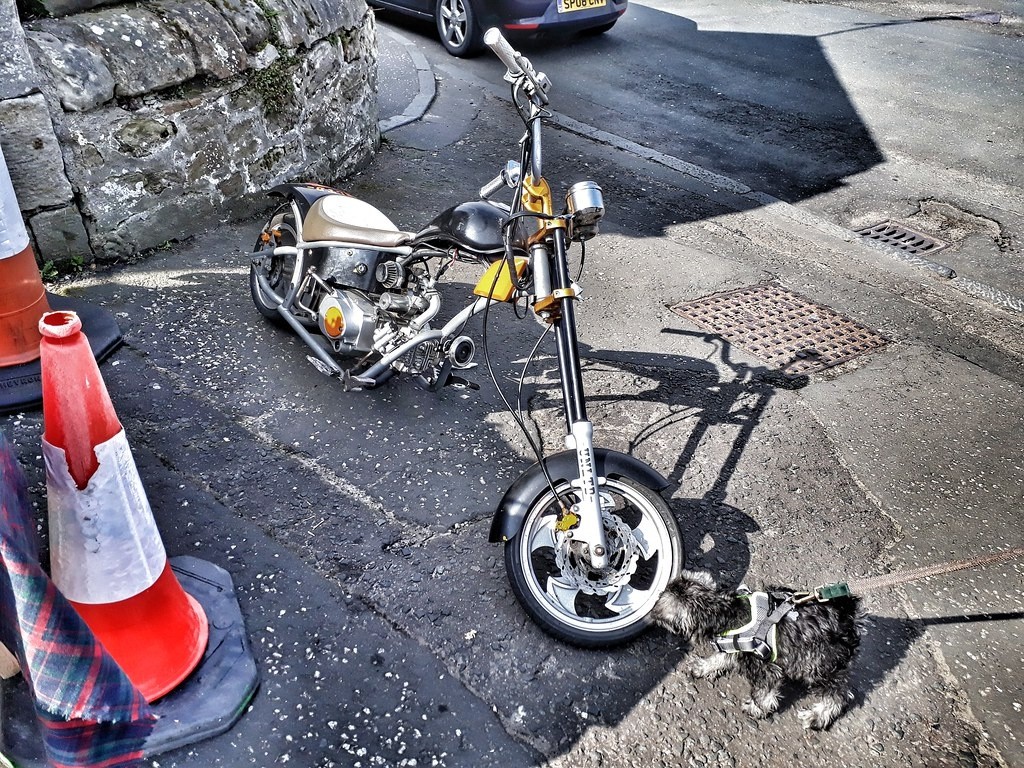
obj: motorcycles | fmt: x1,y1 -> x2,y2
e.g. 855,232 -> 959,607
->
247,27 -> 684,648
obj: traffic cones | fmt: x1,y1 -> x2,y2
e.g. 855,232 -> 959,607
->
0,141 -> 124,413
38,310 -> 261,761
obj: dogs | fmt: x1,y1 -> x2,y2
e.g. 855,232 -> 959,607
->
649,570 -> 875,731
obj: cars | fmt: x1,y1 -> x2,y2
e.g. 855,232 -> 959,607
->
365,0 -> 630,58
